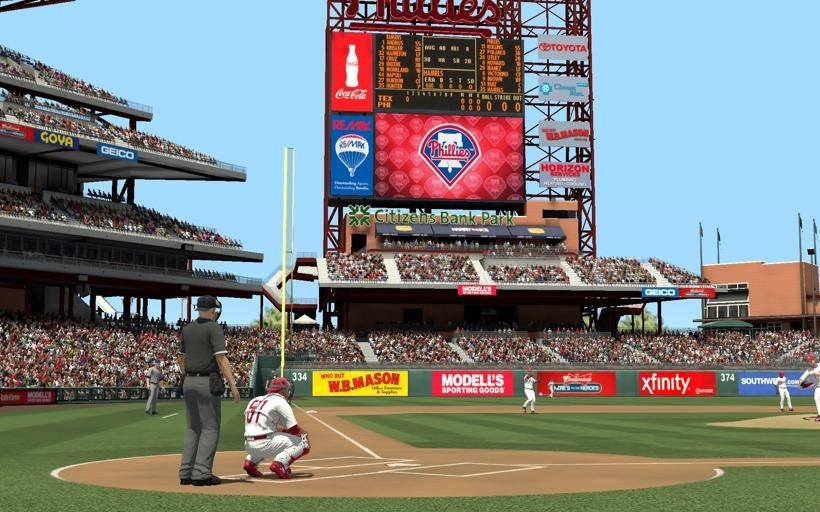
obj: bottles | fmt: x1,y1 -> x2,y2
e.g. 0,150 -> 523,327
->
345,44 -> 359,88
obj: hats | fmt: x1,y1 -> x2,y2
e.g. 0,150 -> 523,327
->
196,296 -> 221,308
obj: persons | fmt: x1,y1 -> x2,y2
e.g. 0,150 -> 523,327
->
243,377 -> 310,479
0,187 -> 243,249
547,378 -> 554,399
523,367 -> 544,414
145,359 -> 164,415
177,297 -> 241,487
799,355 -> 820,421
195,268 -> 236,281
773,371 -> 794,411
0,43 -> 217,165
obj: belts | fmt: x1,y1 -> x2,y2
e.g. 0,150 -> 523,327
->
184,371 -> 208,377
244,435 -> 269,440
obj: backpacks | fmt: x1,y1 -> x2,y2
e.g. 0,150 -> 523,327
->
209,372 -> 225,396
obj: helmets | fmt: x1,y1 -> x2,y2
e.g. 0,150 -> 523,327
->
265,378 -> 291,399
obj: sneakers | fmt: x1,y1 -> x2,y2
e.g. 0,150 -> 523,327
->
192,476 -> 220,485
270,461 -> 292,479
179,477 -> 191,484
244,460 -> 262,477
